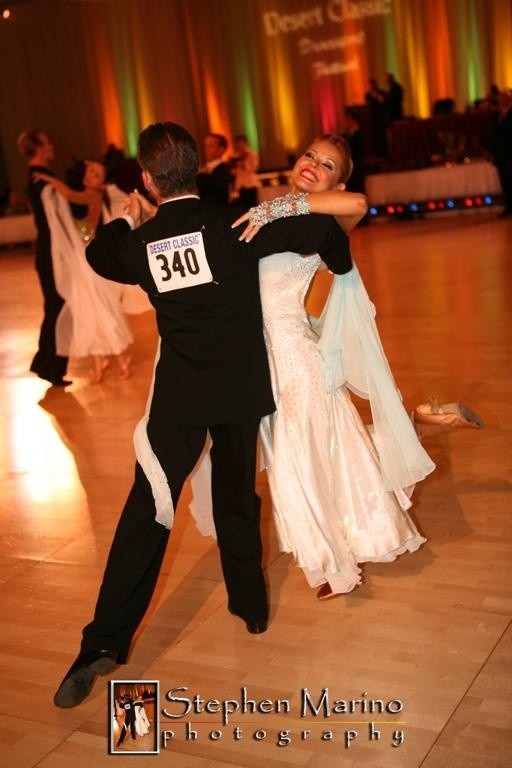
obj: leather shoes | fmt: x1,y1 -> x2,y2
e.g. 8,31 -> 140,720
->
54,637 -> 119,708
38,372 -> 72,385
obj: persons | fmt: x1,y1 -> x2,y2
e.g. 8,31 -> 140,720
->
113,689 -> 155,749
0,128 -> 263,385
53,122 -> 354,711
121,134 -> 485,602
364,74 -> 512,220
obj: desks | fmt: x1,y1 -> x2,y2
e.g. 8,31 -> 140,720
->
365,158 -> 506,224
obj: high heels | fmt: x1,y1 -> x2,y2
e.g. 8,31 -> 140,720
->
317,583 -> 339,600
90,351 -> 138,385
414,396 -> 485,430
